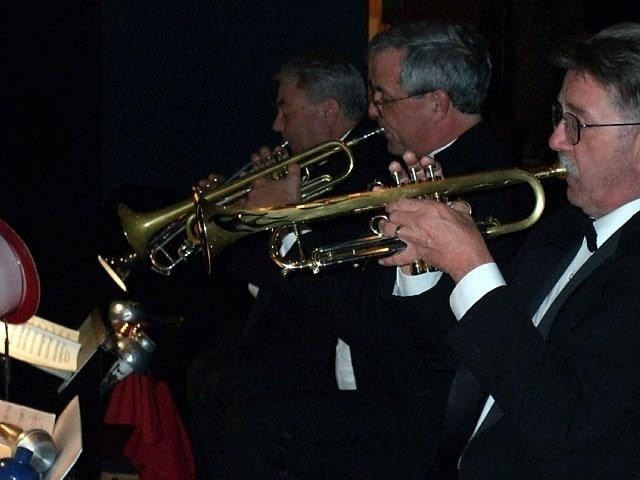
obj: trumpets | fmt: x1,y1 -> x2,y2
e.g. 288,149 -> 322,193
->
185,162 -> 570,281
113,128 -> 385,275
96,139 -> 293,292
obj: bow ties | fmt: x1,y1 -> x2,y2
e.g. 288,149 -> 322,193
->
577,211 -> 597,251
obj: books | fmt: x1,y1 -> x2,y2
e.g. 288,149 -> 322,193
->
0,315 -> 83,373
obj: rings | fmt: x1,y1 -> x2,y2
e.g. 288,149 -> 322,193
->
394,225 -> 405,240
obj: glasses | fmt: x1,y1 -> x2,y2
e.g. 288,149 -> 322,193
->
550,101 -> 640,145
367,80 -> 434,118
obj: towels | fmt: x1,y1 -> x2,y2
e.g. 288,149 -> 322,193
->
103,370 -> 197,480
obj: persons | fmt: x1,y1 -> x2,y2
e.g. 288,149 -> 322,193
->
372,23 -> 640,480
191,47 -> 389,480
239,5 -> 546,480
